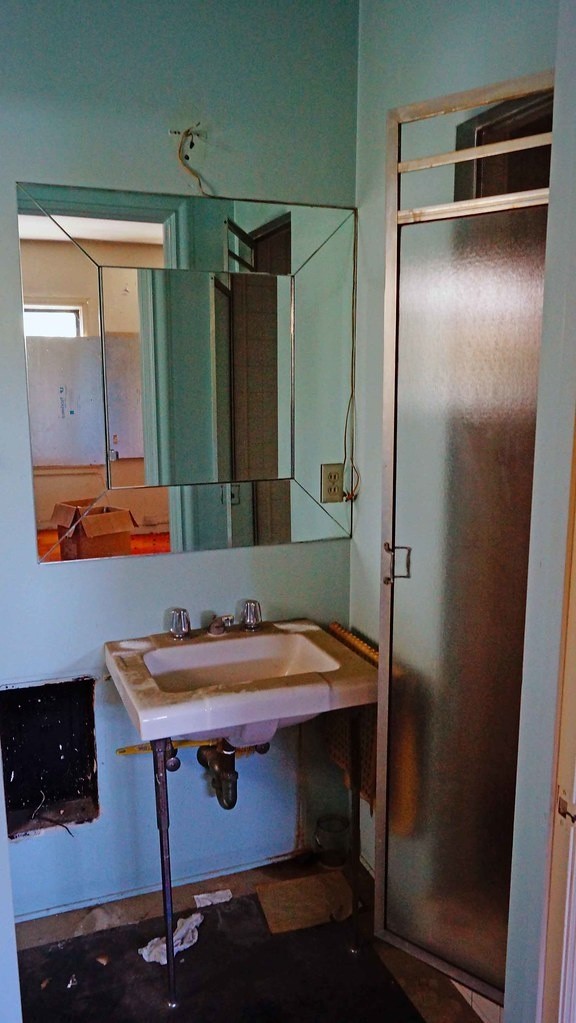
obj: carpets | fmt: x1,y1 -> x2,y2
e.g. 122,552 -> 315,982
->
15,892 -> 428,1022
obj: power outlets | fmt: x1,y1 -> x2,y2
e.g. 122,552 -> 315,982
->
321,464 -> 344,503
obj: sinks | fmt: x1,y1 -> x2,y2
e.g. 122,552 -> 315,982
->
100,630 -> 379,749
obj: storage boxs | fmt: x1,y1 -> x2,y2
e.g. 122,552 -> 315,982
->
51,496 -> 138,560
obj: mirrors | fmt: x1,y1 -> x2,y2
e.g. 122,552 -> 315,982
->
15,183 -> 359,564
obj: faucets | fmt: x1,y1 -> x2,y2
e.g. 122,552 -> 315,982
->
207,613 -> 235,635
